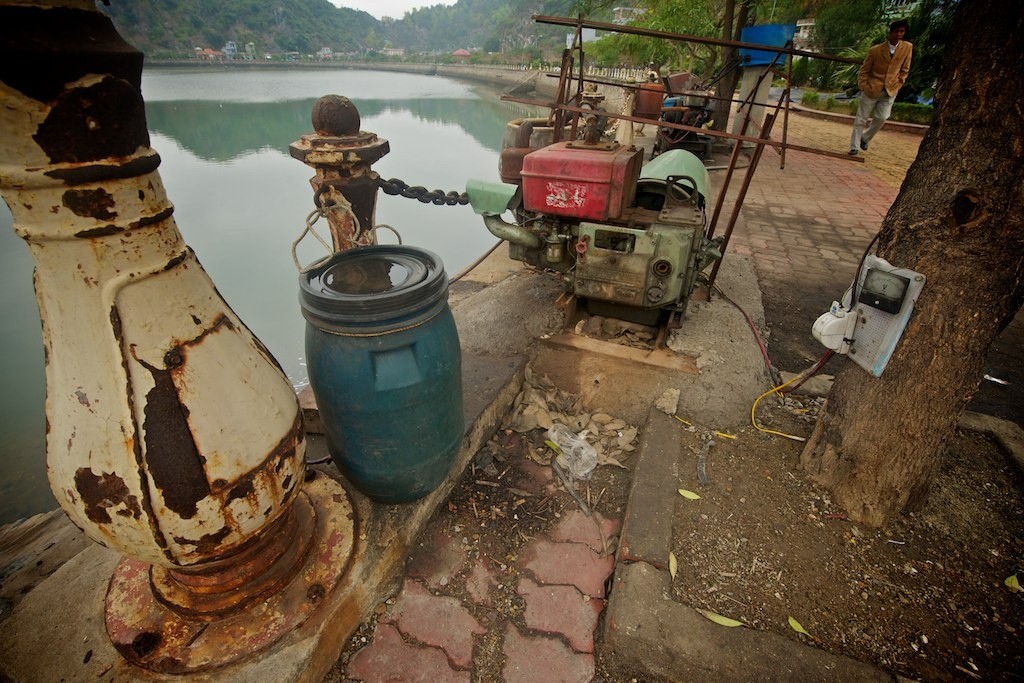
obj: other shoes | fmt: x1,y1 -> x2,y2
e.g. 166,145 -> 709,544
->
847,149 -> 858,155
861,139 -> 868,150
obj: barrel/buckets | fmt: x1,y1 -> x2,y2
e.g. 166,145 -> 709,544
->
297,243 -> 466,505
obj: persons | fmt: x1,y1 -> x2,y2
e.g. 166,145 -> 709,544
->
847,22 -> 913,155
